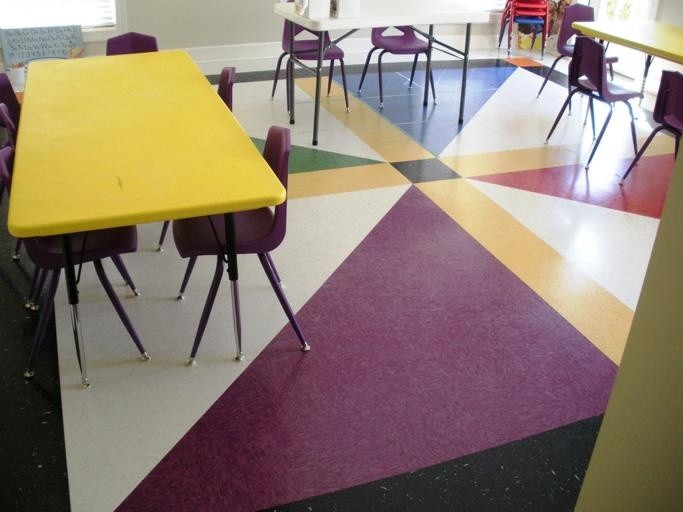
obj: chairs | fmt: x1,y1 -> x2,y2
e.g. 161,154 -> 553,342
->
541,35 -> 645,172
270,0 -> 349,125
0,65 -> 149,377
617,69 -> 682,190
496,0 -> 550,62
106,32 -> 158,54
155,66 -> 313,366
353,24 -> 438,111
532,1 -> 620,118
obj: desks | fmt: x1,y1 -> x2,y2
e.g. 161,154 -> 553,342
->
565,15 -> 682,132
272,0 -> 490,146
6,48 -> 286,388
